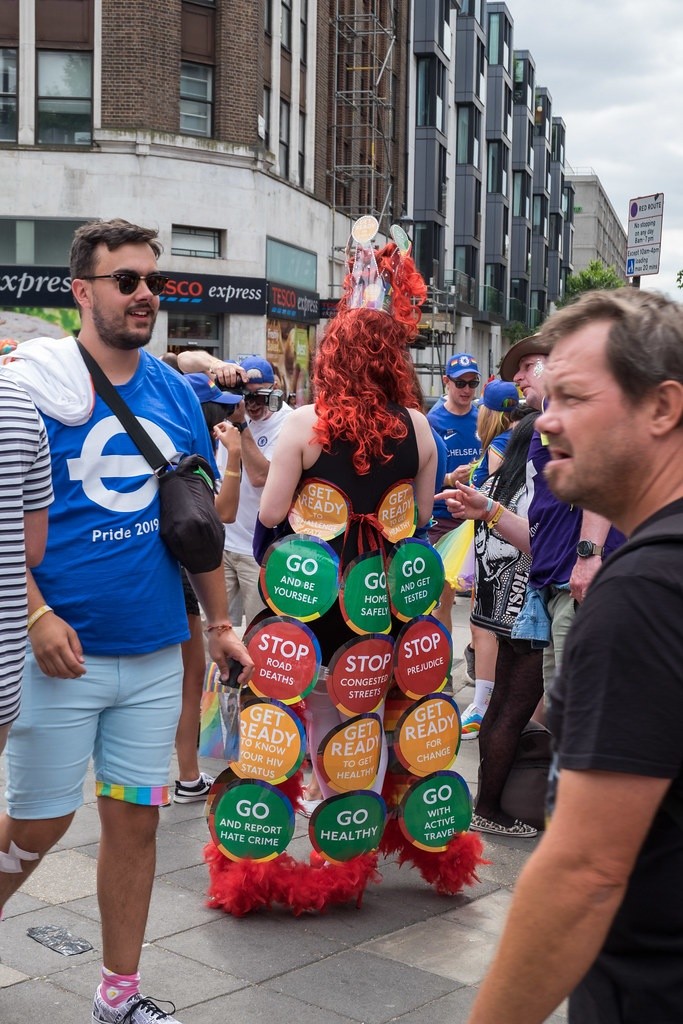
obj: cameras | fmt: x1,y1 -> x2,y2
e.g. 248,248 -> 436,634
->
213,372 -> 283,412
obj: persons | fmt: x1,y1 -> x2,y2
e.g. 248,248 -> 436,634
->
426,330 -> 630,838
157,350 -> 294,804
466,286 -> 683,1024
258,300 -> 438,877
0,217 -> 255,1024
0,374 -> 51,755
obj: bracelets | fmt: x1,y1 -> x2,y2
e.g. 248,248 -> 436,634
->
27,605 -> 53,631
447,473 -> 455,488
479,497 -> 493,520
484,501 -> 500,523
486,504 -> 503,529
209,359 -> 222,374
206,623 -> 232,638
232,421 -> 248,433
224,469 -> 241,478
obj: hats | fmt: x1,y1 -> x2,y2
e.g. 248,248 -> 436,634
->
183,372 -> 244,405
477,379 -> 520,411
500,332 -> 552,382
240,355 -> 274,384
445,352 -> 482,379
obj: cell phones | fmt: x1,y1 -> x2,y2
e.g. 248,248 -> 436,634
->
218,658 -> 243,688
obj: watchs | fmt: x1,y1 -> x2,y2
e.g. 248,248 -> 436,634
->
575,539 -> 605,558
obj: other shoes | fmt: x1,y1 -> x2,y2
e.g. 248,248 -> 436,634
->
469,813 -> 538,838
296,783 -> 324,818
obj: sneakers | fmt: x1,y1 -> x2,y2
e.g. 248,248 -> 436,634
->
464,643 -> 476,686
173,772 -> 215,804
91,984 -> 184,1024
460,703 -> 485,740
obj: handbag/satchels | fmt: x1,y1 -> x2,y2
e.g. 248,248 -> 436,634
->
159,452 -> 226,574
476,719 -> 550,830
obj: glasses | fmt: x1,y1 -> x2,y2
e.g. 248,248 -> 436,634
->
448,375 -> 480,388
82,273 -> 169,296
227,705 -> 235,713
